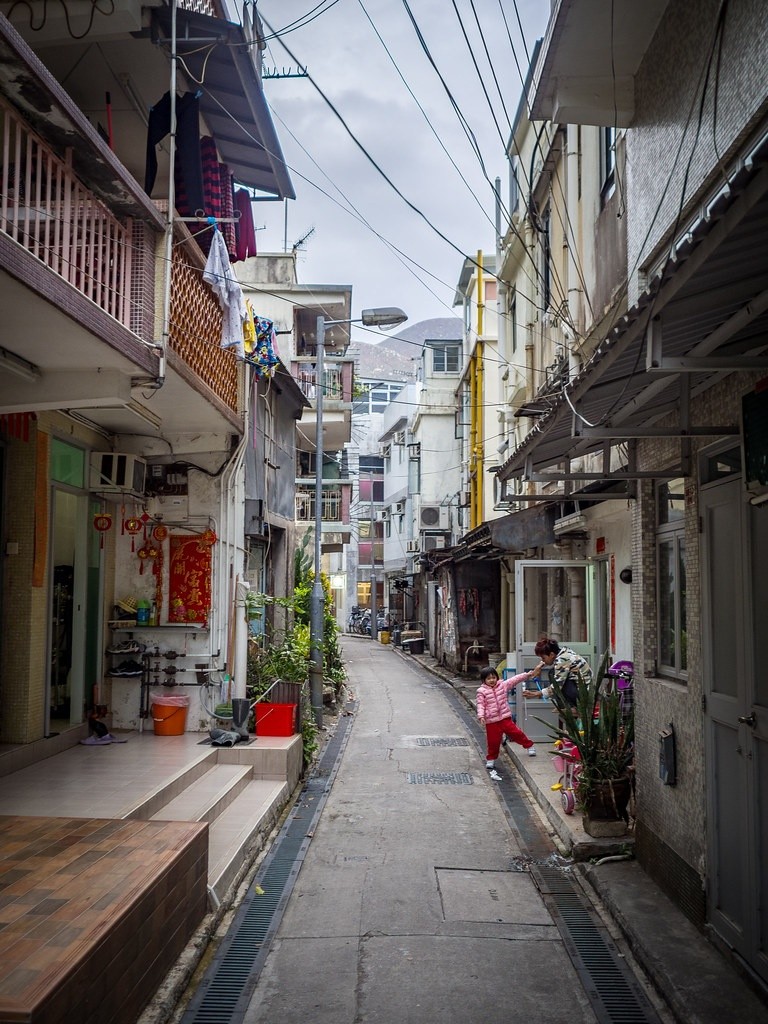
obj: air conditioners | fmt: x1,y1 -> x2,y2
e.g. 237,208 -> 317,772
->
420,536 -> 446,554
406,541 -> 416,552
417,505 -> 451,531
88,451 -> 148,496
376,511 -> 390,522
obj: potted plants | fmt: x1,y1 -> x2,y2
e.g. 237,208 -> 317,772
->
542,651 -> 634,818
389,503 -> 405,515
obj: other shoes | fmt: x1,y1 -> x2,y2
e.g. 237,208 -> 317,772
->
550,707 -> 577,714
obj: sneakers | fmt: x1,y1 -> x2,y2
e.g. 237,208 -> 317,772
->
486,759 -> 495,768
528,746 -> 536,756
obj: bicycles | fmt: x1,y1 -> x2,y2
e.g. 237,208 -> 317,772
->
349,605 -> 388,636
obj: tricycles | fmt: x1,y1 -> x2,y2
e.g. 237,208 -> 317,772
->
550,730 -> 597,815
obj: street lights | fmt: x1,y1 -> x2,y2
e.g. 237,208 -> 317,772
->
348,471 -> 377,639
309,307 -> 409,731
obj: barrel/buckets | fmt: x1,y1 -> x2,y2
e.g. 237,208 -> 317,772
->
378,631 -> 389,644
255,703 -> 297,737
152,696 -> 187,736
489,653 -> 506,669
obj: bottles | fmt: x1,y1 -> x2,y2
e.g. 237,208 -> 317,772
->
138,593 -> 149,627
149,602 -> 158,627
222,674 -> 235,704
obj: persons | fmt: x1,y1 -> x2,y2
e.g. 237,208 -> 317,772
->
523,638 -> 594,714
476,667 -> 536,768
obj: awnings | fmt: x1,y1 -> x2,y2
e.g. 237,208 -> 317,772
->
459,504 -> 551,550
494,97 -> 768,513
